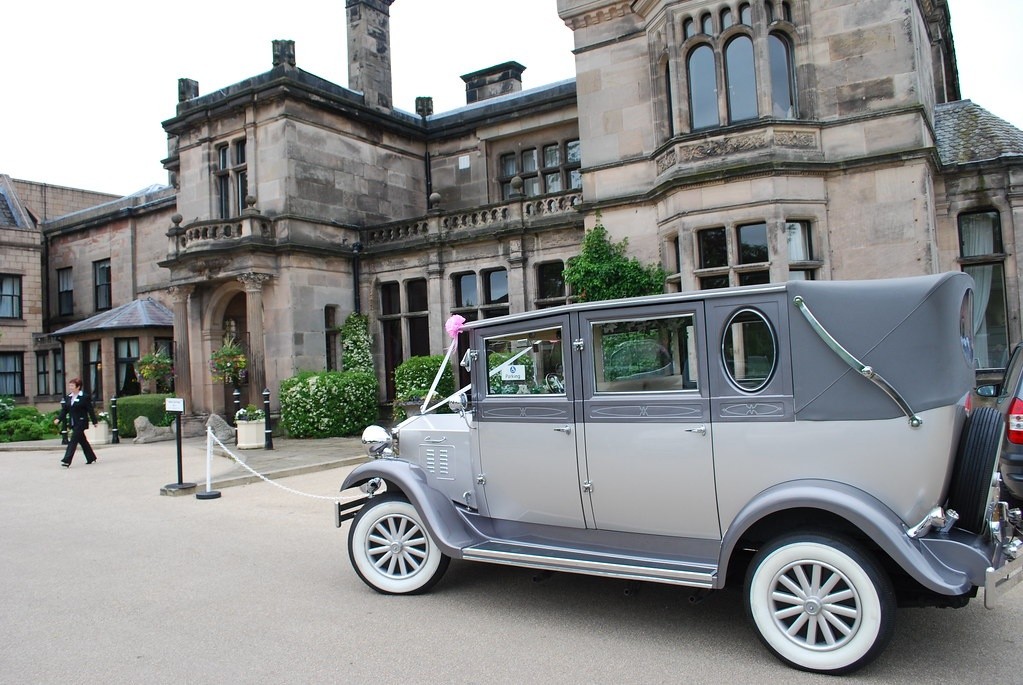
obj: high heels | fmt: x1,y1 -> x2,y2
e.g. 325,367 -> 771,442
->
62,463 -> 69,468
86,459 -> 97,464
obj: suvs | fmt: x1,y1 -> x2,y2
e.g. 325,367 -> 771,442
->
330,273 -> 1023,678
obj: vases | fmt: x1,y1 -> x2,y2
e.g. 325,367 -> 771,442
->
235,418 -> 265,449
84,420 -> 109,445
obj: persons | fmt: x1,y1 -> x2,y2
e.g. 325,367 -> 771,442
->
54,378 -> 97,468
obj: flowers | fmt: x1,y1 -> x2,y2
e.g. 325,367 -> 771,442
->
445,312 -> 466,339
133,344 -> 179,385
88,412 -> 112,428
207,332 -> 250,385
234,404 -> 265,423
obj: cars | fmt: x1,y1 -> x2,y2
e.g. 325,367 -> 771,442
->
975,341 -> 1023,502
